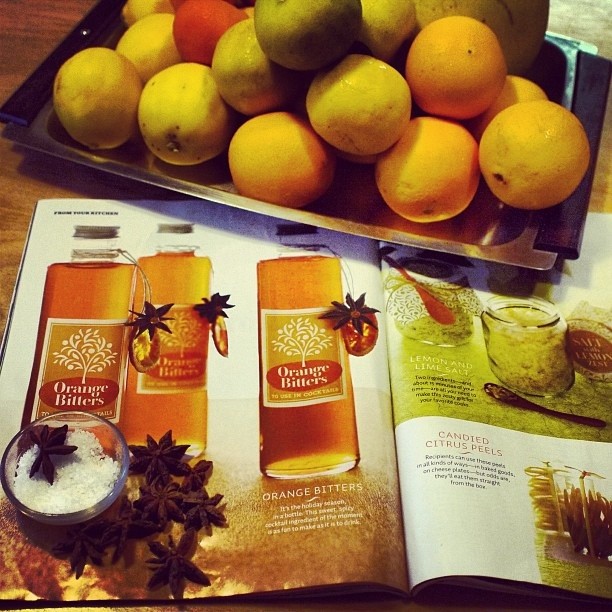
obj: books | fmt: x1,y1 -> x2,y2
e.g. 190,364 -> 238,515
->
0,197 -> 611,611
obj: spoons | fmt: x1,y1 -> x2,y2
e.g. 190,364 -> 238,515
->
484,382 -> 607,430
381,254 -> 455,328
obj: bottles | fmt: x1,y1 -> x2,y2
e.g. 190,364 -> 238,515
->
382,250 -> 480,347
480,295 -> 575,399
118,221 -> 209,458
20,224 -> 137,458
253,222 -> 362,480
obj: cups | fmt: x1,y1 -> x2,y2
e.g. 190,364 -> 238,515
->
0,410 -> 132,527
